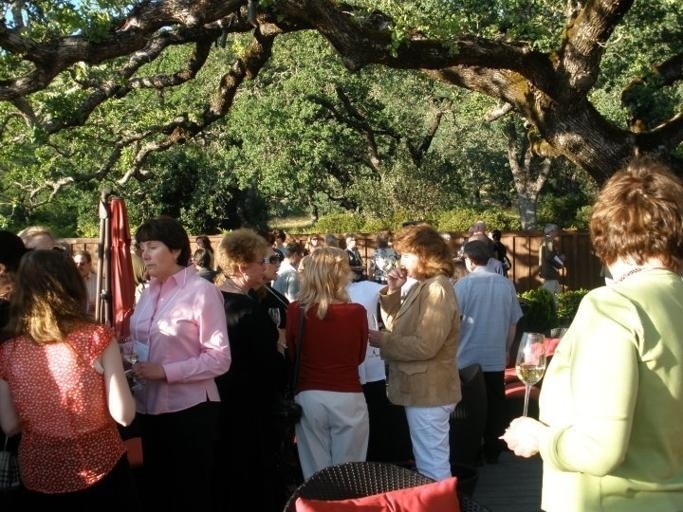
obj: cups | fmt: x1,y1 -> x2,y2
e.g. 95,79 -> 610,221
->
268,307 -> 282,329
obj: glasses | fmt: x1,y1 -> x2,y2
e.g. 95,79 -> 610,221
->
265,255 -> 281,264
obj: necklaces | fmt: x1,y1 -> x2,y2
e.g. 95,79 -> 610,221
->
618,268 -> 642,282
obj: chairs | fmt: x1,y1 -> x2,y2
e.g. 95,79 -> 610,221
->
275,462 -> 491,512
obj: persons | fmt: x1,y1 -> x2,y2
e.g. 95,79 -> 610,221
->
1,222 -> 525,512
541,233 -> 564,295
502,160 -> 683,511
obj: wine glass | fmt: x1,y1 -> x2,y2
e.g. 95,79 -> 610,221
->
125,338 -> 150,391
514,330 -> 547,420
367,314 -> 382,357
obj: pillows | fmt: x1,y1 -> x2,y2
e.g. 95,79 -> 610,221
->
292,476 -> 462,512
504,325 -> 571,410
